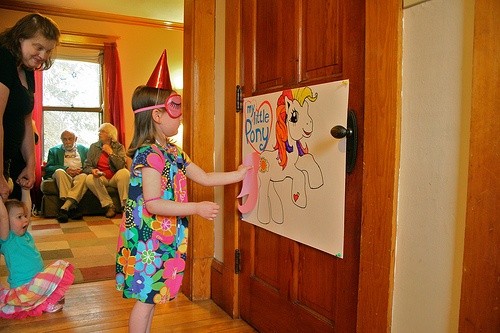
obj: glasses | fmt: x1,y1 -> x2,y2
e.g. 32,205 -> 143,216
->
65,154 -> 76,158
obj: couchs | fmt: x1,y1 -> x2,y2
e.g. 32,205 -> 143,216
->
40,175 -> 122,218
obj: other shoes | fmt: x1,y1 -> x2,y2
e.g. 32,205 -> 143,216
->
56,207 -> 83,223
47,296 -> 65,313
106,207 -> 116,217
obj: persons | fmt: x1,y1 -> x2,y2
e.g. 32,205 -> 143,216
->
45,131 -> 89,223
0,178 -> 75,319
0,13 -> 60,199
116,85 -> 254,333
84,123 -> 130,218
